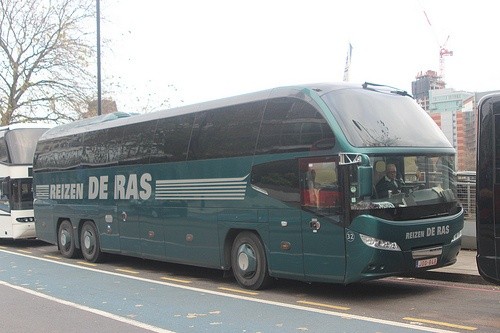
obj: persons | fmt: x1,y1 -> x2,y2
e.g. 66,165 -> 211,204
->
370,161 -> 406,200
306,168 -> 321,190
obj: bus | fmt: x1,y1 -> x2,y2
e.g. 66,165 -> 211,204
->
30,84 -> 466,291
476,92 -> 500,284
0,122 -> 54,242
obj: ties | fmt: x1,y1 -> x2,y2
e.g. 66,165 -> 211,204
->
392,179 -> 397,193
